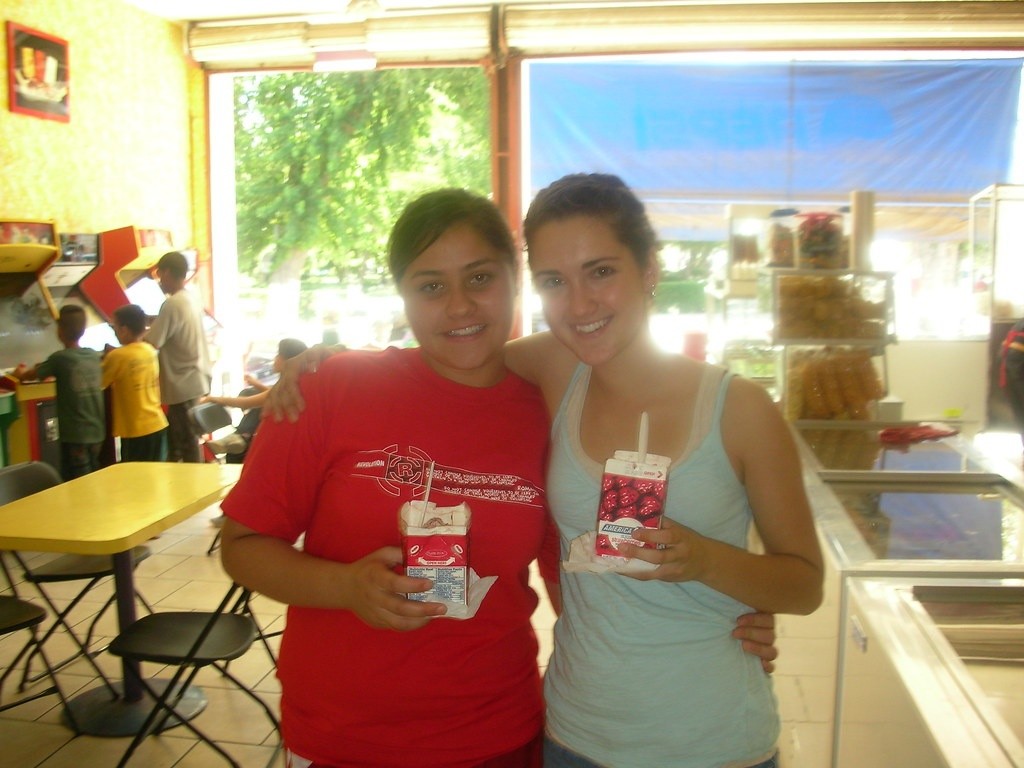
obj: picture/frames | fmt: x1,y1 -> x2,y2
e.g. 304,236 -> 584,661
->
5,20 -> 70,123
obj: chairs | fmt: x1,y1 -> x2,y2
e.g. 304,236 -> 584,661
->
185,401 -> 238,555
0,460 -> 293,768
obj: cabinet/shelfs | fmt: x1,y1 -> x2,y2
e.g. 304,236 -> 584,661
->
723,203 -> 896,422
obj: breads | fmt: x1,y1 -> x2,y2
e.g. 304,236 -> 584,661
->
778,278 -> 888,420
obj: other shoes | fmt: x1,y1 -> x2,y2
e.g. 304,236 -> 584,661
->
211,517 -> 228,527
207,433 -> 246,454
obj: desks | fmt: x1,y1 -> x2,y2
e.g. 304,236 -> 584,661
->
0,462 -> 244,739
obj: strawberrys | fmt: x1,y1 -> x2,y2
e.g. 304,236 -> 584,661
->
597,474 -> 666,522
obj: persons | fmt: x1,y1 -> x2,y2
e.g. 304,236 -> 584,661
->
260,172 -> 826,768
15,304 -> 108,478
102,304 -> 168,463
142,252 -> 212,464
202,336 -> 307,464
219,189 -> 778,768
